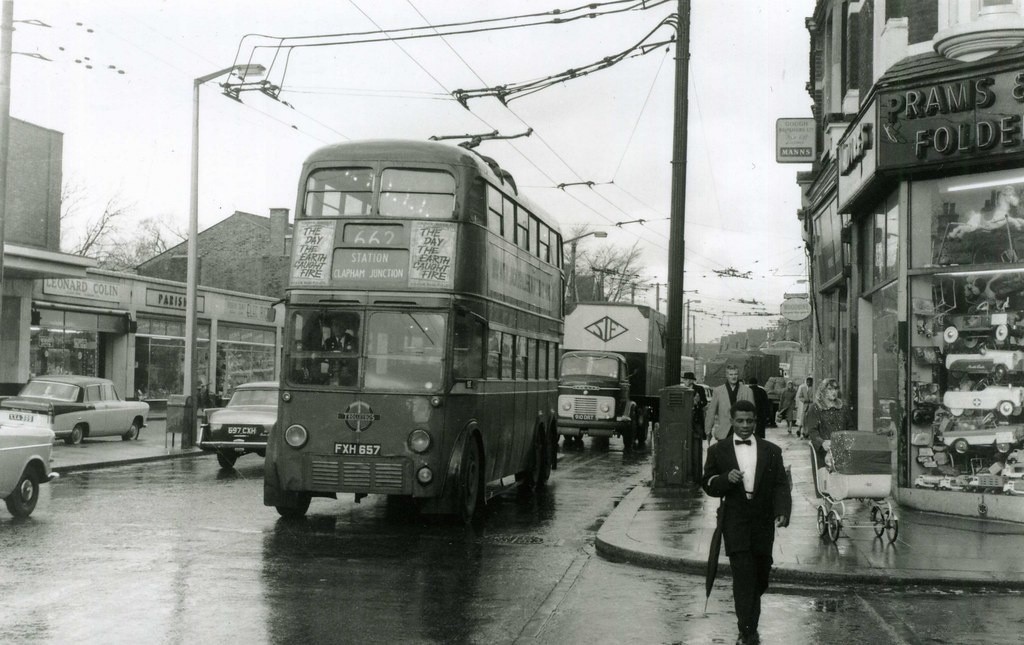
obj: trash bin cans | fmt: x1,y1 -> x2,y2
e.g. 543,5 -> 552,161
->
166,395 -> 193,434
653,385 -> 697,497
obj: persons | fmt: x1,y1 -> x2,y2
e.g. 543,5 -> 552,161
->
779,382 -> 796,433
807,378 -> 856,512
701,401 -> 793,645
794,377 -> 814,438
681,372 -> 707,410
749,378 -> 767,439
705,365 -> 756,442
304,316 -> 357,367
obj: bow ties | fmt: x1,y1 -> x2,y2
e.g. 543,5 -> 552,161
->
735,440 -> 752,446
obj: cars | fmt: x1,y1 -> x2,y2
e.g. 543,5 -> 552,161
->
0,394 -> 61,517
12,372 -> 150,445
200,380 -> 279,469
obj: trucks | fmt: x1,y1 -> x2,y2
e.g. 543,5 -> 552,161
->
554,300 -> 667,457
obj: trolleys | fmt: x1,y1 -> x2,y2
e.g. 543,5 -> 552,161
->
802,438 -> 899,544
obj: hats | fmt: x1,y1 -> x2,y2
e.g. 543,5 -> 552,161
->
681,372 -> 696,380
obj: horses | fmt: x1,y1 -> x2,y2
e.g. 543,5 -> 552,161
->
945,185 -> 1024,240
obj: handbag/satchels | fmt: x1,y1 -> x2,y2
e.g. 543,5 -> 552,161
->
776,412 -> 783,423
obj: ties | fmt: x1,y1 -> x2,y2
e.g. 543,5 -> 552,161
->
337,336 -> 342,350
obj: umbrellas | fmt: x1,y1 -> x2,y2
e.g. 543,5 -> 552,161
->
705,476 -> 726,613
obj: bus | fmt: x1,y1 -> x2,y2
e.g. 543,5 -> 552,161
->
262,127 -> 565,527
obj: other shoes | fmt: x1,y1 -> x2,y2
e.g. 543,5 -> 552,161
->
736,633 -> 760,645
788,429 -> 791,434
796,431 -> 800,437
802,432 -> 806,438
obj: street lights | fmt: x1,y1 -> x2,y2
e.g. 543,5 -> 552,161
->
181,63 -> 266,450
